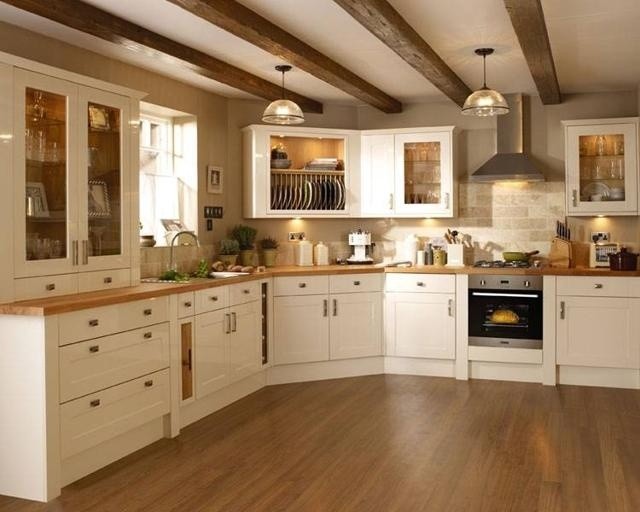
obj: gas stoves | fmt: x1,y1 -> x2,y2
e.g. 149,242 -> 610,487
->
467,260 -> 543,290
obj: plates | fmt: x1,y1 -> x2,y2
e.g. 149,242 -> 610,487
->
210,271 -> 250,279
271,145 -> 344,210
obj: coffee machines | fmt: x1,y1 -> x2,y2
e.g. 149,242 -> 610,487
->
348,231 -> 373,264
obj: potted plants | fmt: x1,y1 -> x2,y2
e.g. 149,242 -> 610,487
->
233,223 -> 258,267
216,238 -> 240,266
260,236 -> 280,267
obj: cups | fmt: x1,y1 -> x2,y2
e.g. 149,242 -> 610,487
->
330,254 -> 346,265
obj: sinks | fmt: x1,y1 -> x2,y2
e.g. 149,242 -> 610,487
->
206,272 -> 250,279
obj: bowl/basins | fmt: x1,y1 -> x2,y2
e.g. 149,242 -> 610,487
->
582,182 -> 624,201
27,233 -> 65,260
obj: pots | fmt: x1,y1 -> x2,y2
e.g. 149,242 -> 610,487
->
503,250 -> 539,261
608,248 -> 639,270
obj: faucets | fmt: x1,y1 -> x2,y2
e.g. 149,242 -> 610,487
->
170,230 -> 201,269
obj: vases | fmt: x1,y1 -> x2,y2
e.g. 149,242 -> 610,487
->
139,234 -> 154,247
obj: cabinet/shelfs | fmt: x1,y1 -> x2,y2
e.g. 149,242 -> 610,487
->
559,119 -> 640,217
395,126 -> 459,219
360,129 -> 395,220
168,271 -> 273,439
0,286 -> 180,503
555,269 -> 640,389
240,124 -> 360,219
1,51 -> 149,305
270,267 -> 385,387
384,266 -> 469,381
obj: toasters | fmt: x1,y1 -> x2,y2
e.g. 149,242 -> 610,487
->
590,242 -> 620,269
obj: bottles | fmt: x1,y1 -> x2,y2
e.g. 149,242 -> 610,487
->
417,243 -> 445,267
179,242 -> 192,273
88,222 -> 107,257
581,135 -> 624,179
26,188 -> 43,218
404,143 -> 441,204
140,235 -> 157,247
27,91 -> 61,164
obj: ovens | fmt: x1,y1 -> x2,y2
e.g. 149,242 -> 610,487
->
468,288 -> 543,350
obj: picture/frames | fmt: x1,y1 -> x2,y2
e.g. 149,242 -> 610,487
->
207,165 -> 223,194
88,104 -> 112,131
87,176 -> 112,220
25,182 -> 50,217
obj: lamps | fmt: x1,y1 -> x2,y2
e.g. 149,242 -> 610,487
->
262,64 -> 305,124
461,47 -> 511,116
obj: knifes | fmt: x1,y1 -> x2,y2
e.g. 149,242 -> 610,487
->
556,216 -> 571,241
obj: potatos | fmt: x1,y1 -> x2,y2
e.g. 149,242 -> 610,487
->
211,260 -> 254,273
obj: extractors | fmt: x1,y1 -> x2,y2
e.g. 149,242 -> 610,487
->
471,93 -> 547,183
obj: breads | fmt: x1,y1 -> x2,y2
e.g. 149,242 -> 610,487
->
490,309 -> 521,324
